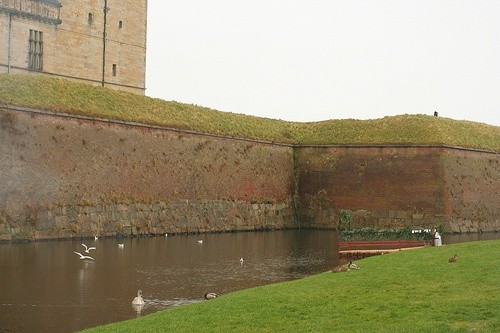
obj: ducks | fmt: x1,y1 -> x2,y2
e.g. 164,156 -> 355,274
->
132,290 -> 144,304
332,260 -> 360,273
204,293 -> 217,299
448,254 -> 457,263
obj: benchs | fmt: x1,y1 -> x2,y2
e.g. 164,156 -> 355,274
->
338,241 -> 426,259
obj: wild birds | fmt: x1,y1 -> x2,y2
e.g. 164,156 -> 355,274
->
72,251 -> 95,260
95,236 -> 98,240
81,244 -> 96,253
197,240 -> 202,243
